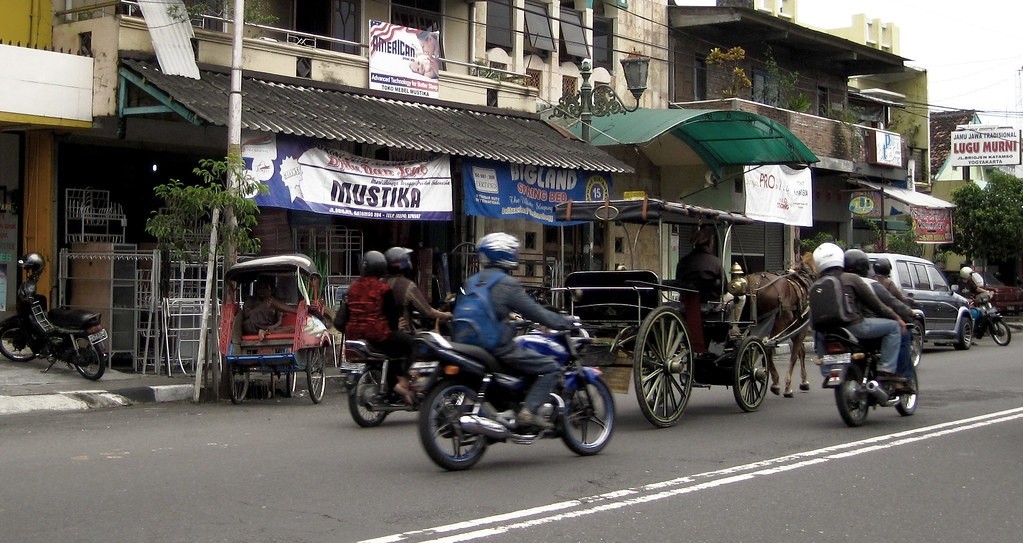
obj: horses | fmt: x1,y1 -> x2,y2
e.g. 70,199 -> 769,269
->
744,251 -> 820,398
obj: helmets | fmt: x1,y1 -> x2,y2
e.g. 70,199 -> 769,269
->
360,251 -> 388,279
873,258 -> 892,276
844,249 -> 869,273
476,231 -> 520,270
384,246 -> 413,269
812,242 -> 844,275
21,252 -> 45,275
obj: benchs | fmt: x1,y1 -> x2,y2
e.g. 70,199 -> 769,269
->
240,311 -> 298,353
660,280 -> 723,313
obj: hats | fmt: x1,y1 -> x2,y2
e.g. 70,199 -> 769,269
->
959,267 -> 974,279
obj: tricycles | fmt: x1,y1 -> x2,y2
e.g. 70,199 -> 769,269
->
220,253 -> 332,406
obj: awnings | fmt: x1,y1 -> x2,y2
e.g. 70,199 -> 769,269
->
847,177 -> 958,250
116,56 -> 635,174
537,106 -> 821,181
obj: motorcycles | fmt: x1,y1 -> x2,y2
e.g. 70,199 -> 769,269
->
417,288 -> 617,473
962,288 -> 1011,346
339,296 -> 456,428
814,290 -> 925,428
0,254 -> 109,381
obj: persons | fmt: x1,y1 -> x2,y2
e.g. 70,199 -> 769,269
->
813,242 -> 923,388
409,31 -> 438,78
454,232 -> 579,427
380,247 -> 454,335
950,267 -> 996,345
676,228 -> 746,336
242,273 -> 297,334
333,251 -> 414,405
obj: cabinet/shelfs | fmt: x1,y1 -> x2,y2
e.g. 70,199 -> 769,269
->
49,234 -> 226,375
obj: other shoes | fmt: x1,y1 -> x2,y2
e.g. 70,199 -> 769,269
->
393,383 -> 414,405
517,408 -> 556,428
893,386 -> 917,395
878,373 -> 907,383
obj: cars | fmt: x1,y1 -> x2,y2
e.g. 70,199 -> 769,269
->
942,268 -> 1023,318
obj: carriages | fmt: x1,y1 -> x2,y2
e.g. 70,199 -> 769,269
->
556,250 -> 819,429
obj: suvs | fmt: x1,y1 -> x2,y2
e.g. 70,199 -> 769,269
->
866,252 -> 974,351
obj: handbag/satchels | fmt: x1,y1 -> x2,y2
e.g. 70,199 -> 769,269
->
334,289 -> 349,333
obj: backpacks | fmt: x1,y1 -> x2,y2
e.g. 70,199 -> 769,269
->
808,273 -> 859,332
452,273 -> 505,349
